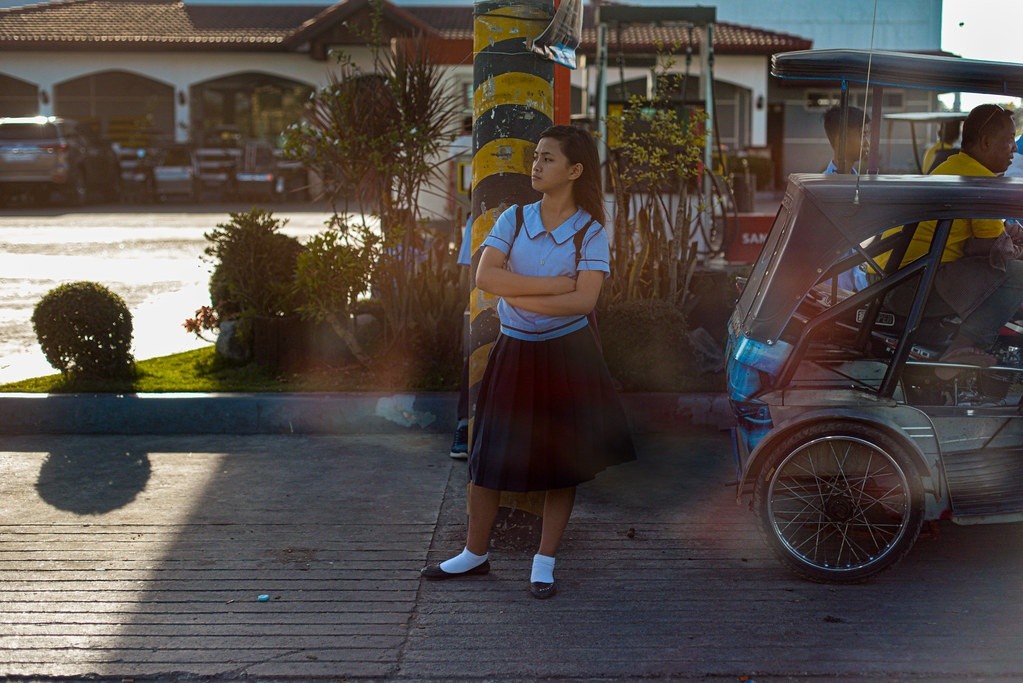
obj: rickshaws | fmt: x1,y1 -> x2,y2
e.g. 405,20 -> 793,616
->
722,47 -> 1023,584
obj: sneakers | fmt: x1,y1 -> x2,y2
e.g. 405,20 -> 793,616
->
450,425 -> 469,460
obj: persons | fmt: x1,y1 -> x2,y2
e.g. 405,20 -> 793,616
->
449,178 -> 474,460
421,124 -> 612,598
865,102 -> 1023,380
923,119 -> 961,176
816,102 -> 872,293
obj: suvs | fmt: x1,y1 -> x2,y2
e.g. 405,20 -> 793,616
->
0,116 -> 103,206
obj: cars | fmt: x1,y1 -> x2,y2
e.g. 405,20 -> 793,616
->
109,136 -> 306,207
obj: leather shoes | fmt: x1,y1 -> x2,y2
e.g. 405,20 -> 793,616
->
530,571 -> 558,599
421,558 -> 491,579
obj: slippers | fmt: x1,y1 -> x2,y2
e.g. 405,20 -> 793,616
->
935,348 -> 997,381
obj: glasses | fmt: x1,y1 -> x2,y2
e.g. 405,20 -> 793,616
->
978,104 -> 1004,132
